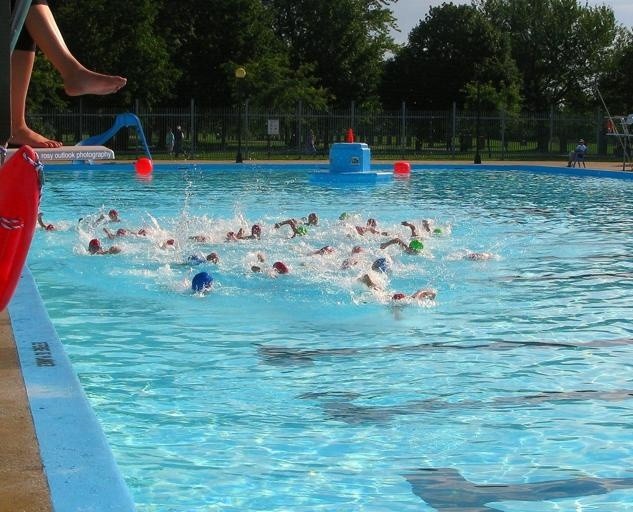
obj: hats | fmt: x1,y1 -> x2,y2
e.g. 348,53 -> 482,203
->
433,228 -> 444,235
117,229 -> 126,237
372,258 -> 391,272
88,239 -> 101,249
339,212 -> 351,221
109,209 -> 118,217
272,262 -> 288,275
408,239 -> 425,253
47,223 -> 54,231
296,225 -> 307,235
391,294 -> 405,300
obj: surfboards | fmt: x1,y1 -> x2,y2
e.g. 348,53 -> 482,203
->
1,146 -> 44,309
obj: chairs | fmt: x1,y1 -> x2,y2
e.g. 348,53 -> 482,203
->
571,148 -> 587,168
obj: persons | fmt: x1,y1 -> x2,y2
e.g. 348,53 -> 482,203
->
358,258 -> 435,300
173,125 -> 189,160
565,139 -> 587,167
165,129 -> 174,158
10,0 -> 130,149
306,128 -> 317,158
35,207 -> 495,274
